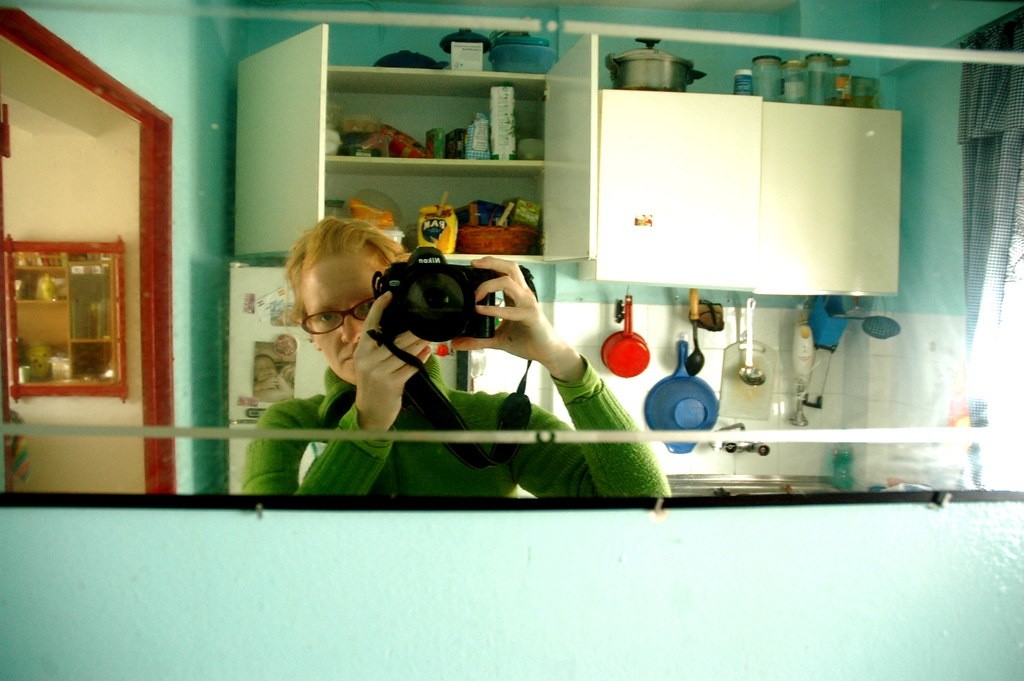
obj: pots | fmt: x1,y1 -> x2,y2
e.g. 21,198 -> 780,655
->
644,341 -> 719,453
605,38 -> 707,92
372,50 -> 450,69
600,295 -> 651,378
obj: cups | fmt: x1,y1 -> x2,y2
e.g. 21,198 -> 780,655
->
19,366 -> 31,383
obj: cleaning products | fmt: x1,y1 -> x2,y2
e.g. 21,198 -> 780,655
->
833,443 -> 854,488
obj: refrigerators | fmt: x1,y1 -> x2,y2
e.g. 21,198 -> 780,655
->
227,262 -> 457,505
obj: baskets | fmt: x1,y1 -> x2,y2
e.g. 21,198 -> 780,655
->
457,206 -> 539,254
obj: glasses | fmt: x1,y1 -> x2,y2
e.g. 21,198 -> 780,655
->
301,297 -> 377,334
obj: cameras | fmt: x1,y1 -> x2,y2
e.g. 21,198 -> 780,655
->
382,246 -> 498,343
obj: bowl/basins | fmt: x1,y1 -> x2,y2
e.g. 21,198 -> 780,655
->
489,36 -> 560,73
324,102 -> 384,157
455,226 -> 538,254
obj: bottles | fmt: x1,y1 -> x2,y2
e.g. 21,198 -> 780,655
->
42,273 -> 56,300
731,53 -> 853,108
25,339 -> 54,380
325,199 -> 346,220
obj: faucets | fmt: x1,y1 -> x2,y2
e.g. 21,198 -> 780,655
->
712,422 -> 770,455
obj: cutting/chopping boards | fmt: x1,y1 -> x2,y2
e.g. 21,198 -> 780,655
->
718,339 -> 778,421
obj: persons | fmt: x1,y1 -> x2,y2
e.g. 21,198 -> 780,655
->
241,215 -> 671,499
253,353 -> 294,401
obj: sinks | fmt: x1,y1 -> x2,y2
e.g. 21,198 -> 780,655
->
665,473 -> 788,494
788,474 -> 838,495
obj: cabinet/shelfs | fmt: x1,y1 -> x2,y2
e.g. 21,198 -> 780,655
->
233,24 -> 601,264
599,93 -> 902,294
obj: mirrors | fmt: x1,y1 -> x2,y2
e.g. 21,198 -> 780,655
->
5,232 -> 128,403
0,0 -> 1024,512
